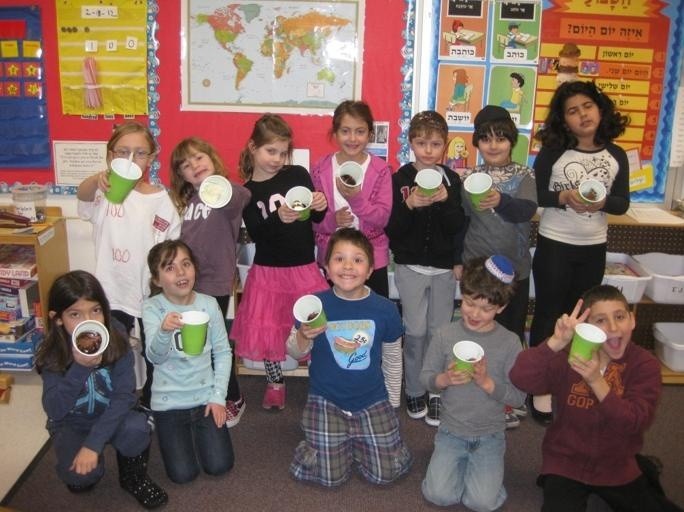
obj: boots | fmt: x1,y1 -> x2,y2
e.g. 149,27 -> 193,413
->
116,448 -> 169,510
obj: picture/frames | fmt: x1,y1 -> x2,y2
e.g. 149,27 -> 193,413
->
179,1 -> 364,117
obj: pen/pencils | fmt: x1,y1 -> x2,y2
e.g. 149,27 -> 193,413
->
83,56 -> 102,111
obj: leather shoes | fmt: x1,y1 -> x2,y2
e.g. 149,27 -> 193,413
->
529,394 -> 553,426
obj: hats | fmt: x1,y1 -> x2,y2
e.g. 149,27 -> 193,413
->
474,104 -> 512,124
559,43 -> 580,57
484,254 -> 515,284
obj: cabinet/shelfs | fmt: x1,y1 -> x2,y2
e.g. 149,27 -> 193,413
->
1,210 -> 68,371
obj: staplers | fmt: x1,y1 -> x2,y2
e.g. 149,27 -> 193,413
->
0,212 -> 31,228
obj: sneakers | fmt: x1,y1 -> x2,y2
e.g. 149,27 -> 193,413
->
425,391 -> 441,427
648,456 -> 662,474
504,404 -> 528,429
225,392 -> 247,429
405,391 -> 429,420
262,378 -> 286,410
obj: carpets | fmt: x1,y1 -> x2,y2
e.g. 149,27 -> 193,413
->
0,374 -> 684,511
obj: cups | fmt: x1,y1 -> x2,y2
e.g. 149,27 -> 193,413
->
450,339 -> 485,372
198,174 -> 233,208
415,167 -> 444,195
578,178 -> 606,207
291,294 -> 329,328
568,321 -> 608,367
178,311 -> 212,361
463,170 -> 493,213
103,157 -> 144,203
337,160 -> 364,189
70,319 -> 112,355
285,185 -> 314,223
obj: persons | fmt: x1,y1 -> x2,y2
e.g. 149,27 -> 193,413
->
138,238 -> 235,486
454,104 -> 534,345
508,283 -> 684,511
228,111 -> 330,417
420,256 -> 526,509
74,122 -> 182,429
528,82 -> 629,352
284,228 -> 410,487
165,138 -> 250,431
307,101 -> 395,294
37,268 -> 171,508
387,110 -> 470,427
